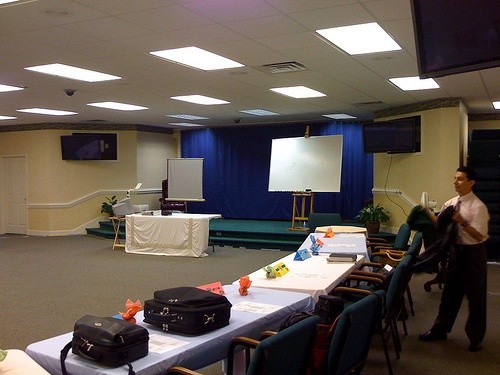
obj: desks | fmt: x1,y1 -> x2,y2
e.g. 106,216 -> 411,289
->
109,214 -> 222,258
232,253 -> 365,296
25,285 -> 314,375
297,233 -> 374,272
314,226 -> 369,238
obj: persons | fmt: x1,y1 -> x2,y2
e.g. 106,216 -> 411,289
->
417,167 -> 490,353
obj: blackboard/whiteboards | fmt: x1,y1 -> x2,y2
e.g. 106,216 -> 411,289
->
268,134 -> 344,192
164,158 -> 205,201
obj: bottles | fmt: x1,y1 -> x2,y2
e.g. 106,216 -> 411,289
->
303,221 -> 306,228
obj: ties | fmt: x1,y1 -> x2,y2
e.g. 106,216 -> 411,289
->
453,200 -> 461,212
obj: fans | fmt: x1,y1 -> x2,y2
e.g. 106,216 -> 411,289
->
421,192 -> 437,211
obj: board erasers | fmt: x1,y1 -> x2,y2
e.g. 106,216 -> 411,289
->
305,188 -> 312,193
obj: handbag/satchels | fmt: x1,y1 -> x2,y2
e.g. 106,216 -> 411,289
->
144,286 -> 232,337
379,249 -> 408,266
279,294 -> 346,369
60,313 -> 149,375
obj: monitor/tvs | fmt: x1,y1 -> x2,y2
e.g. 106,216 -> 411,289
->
60,133 -> 117,160
409,0 -> 500,80
112,198 -> 134,218
363,119 -> 416,154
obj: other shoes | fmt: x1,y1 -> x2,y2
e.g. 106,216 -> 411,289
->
419,328 -> 448,342
468,342 -> 482,352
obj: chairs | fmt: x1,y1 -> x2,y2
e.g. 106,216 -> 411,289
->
167,224 -> 424,375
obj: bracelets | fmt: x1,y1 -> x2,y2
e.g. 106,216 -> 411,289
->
460,221 -> 468,230
